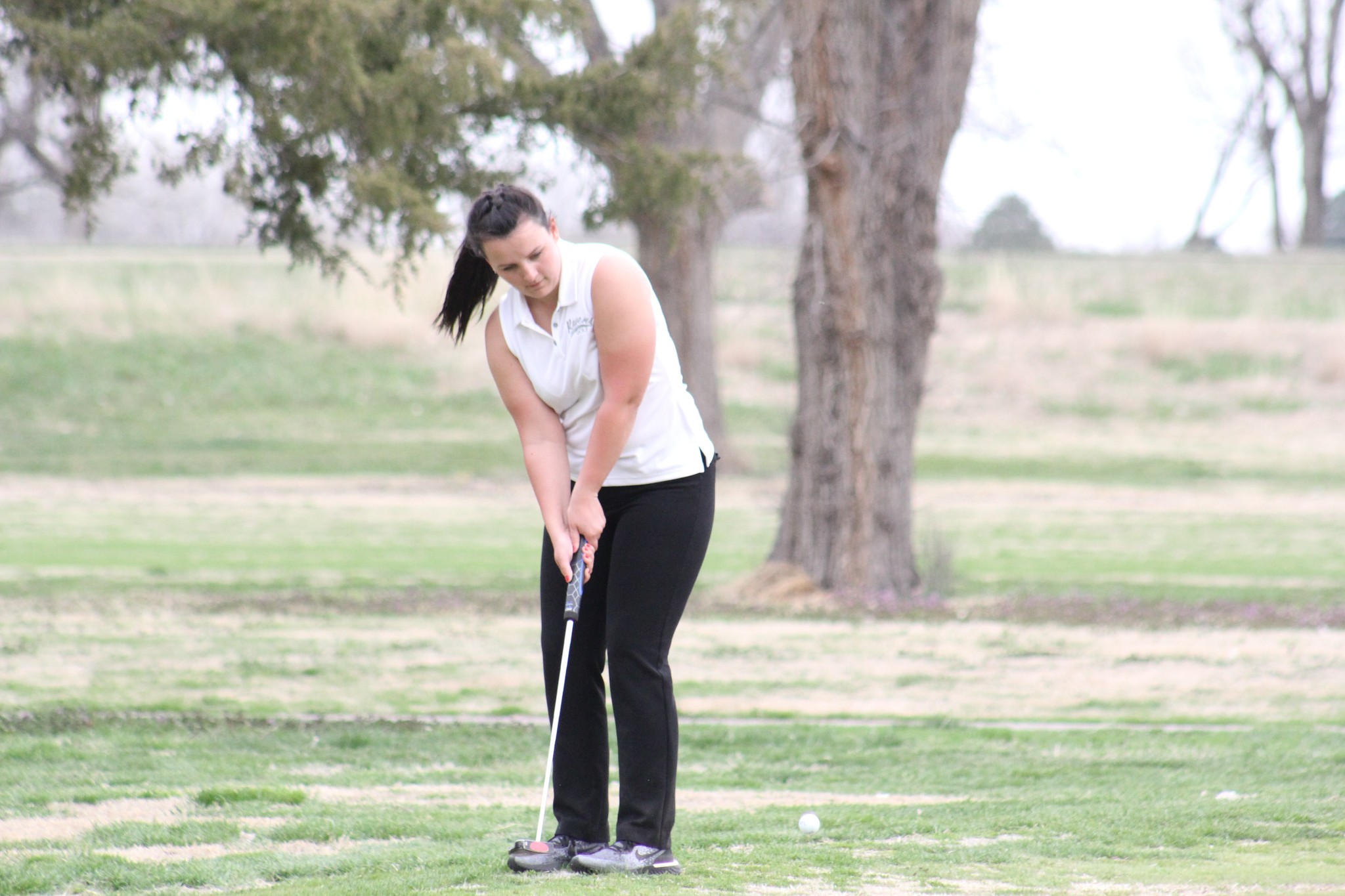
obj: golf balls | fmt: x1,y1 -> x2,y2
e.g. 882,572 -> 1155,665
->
798,813 -> 820,835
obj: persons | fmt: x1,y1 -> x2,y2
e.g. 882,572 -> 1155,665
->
435,189 -> 720,879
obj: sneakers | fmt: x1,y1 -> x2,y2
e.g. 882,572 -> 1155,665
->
570,841 -> 682,876
507,836 -> 609,873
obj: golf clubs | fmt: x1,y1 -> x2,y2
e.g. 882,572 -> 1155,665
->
509,539 -> 588,858
